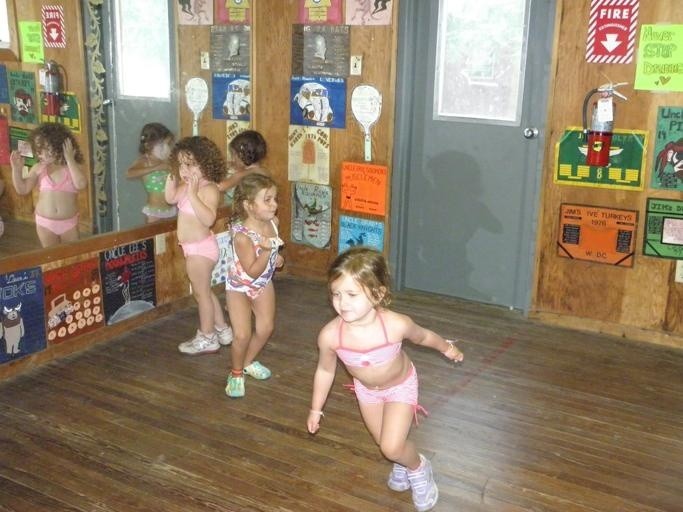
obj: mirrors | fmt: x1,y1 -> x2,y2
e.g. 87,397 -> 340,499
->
0,0 -> 256,259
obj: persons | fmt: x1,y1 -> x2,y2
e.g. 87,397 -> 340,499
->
125,122 -> 178,223
307,246 -> 464,511
164,135 -> 234,356
9,123 -> 88,247
220,129 -> 271,193
224,173 -> 285,399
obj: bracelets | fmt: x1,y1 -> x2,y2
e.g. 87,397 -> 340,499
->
310,407 -> 325,420
443,337 -> 457,356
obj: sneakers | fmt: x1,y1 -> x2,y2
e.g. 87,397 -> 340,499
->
178,327 -> 233,354
225,371 -> 246,397
388,463 -> 410,491
243,361 -> 271,380
406,456 -> 438,511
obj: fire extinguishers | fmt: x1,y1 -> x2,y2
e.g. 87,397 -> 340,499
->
583,82 -> 630,166
39,57 -> 68,115
